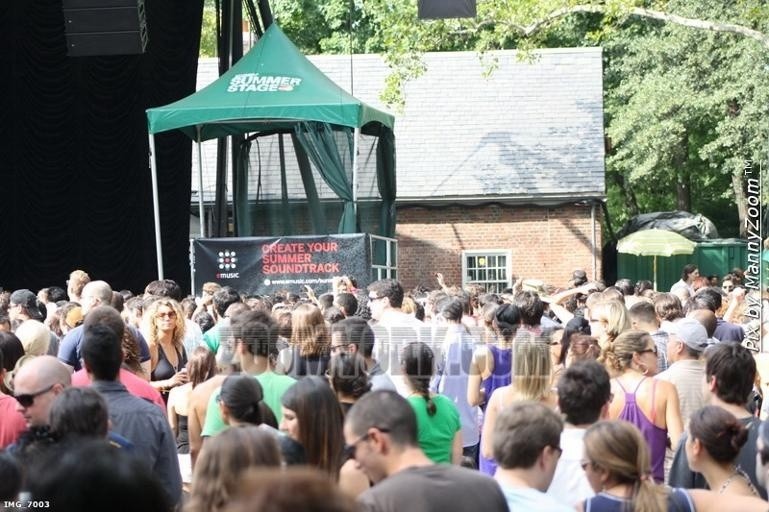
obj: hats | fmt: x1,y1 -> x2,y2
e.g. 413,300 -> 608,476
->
661,316 -> 710,354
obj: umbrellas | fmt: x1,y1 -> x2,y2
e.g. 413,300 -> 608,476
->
616,227 -> 696,292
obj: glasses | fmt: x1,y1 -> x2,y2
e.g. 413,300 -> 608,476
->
11,383 -> 65,408
588,315 -> 608,324
342,426 -> 391,458
580,457 -> 598,470
627,344 -> 658,359
603,391 -> 615,403
202,289 -> 214,295
367,293 -> 389,303
154,310 -> 177,322
215,393 -> 224,405
328,343 -> 358,353
65,280 -> 74,286
549,442 -> 564,459
723,285 -> 734,290
6,302 -> 21,310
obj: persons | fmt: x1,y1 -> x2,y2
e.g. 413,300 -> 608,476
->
0,263 -> 769,512
743,236 -> 769,293
